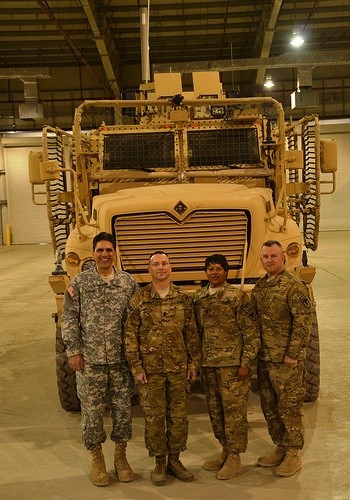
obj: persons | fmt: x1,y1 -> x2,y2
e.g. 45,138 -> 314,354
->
251,240 -> 312,477
122,251 -> 202,486
61,231 -> 139,486
192,254 -> 262,480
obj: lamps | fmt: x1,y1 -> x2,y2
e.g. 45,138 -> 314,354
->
289,3 -> 305,48
264,70 -> 274,89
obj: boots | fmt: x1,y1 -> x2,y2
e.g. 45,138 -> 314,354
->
113,443 -> 135,483
89,444 -> 110,486
166,454 -> 194,482
150,454 -> 168,485
217,451 -> 241,479
275,452 -> 301,477
257,447 -> 285,468
201,451 -> 228,469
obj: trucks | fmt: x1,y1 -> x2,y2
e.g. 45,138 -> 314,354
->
29,67 -> 335,413
